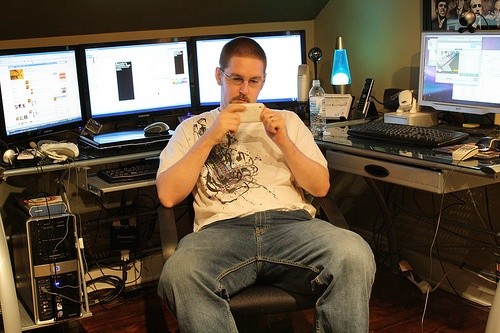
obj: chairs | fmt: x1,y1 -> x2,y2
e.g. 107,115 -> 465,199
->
157,194 -> 350,333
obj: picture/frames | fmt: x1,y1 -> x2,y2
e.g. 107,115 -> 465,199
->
422,0 -> 500,32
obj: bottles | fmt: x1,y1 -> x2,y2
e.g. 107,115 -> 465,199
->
308,80 -> 327,133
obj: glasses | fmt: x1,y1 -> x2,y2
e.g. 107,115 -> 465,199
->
221,69 -> 265,87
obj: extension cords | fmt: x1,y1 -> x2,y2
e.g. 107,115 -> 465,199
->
398,260 -> 430,294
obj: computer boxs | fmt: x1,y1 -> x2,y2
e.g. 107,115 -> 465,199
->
6,201 -> 83,325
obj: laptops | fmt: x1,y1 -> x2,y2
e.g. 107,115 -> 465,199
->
79,128 -> 175,149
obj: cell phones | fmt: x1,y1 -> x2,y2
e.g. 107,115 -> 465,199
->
234,103 -> 264,122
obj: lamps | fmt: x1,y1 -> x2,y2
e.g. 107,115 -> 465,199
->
331,37 -> 352,94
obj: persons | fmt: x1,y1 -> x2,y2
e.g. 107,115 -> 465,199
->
432,0 -> 448,30
470,0 -> 482,15
449,0 -> 468,18
151,37 -> 376,333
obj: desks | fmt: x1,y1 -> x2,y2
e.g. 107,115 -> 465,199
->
0,134 -> 500,333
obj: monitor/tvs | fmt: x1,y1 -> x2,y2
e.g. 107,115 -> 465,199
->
418,30 -> 500,115
0,29 -> 308,145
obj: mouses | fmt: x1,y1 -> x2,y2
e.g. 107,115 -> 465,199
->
144,122 -> 169,133
475,137 -> 500,151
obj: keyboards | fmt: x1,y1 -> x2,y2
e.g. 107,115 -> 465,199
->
349,121 -> 470,147
98,162 -> 160,184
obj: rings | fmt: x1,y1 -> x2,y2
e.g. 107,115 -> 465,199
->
269,116 -> 274,122
